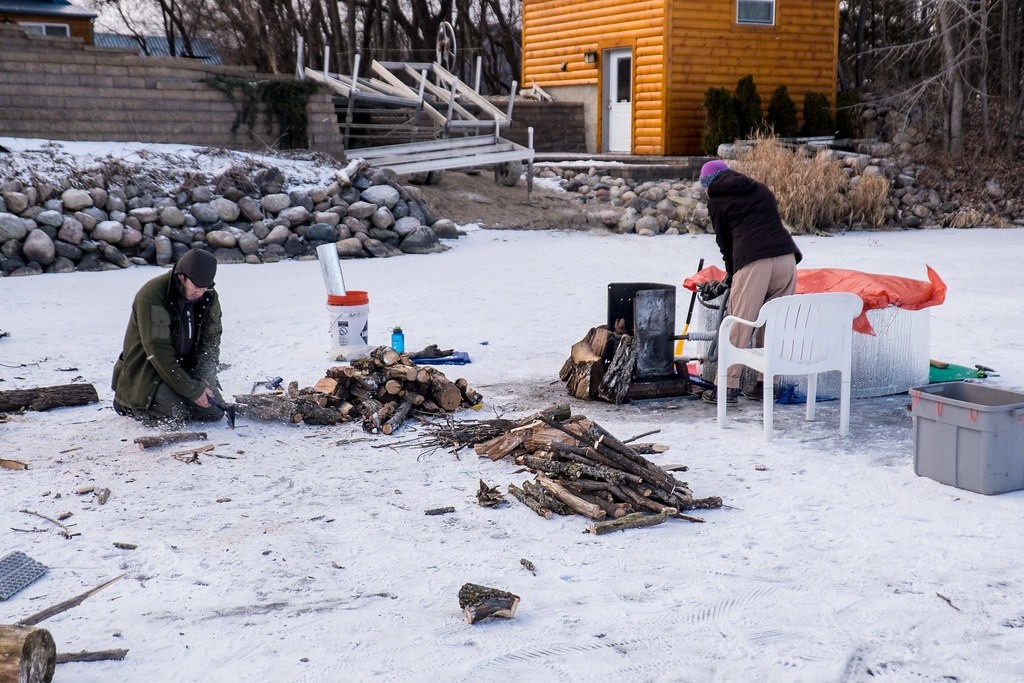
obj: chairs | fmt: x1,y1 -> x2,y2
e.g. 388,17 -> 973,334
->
716,292 -> 863,441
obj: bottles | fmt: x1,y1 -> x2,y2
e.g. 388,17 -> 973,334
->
387,326 -> 404,356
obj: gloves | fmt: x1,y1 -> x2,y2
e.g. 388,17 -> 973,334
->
408,344 -> 455,360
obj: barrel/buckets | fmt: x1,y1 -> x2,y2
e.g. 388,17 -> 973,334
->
327,290 -> 370,347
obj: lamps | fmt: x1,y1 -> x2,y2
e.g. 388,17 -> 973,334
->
584,49 -> 598,63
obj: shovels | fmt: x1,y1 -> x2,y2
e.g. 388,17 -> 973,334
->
676,259 -> 704,356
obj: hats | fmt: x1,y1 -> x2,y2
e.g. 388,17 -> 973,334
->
174,248 -> 217,289
699,159 -> 730,186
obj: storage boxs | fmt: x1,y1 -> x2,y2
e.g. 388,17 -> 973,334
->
908,381 -> 1024,496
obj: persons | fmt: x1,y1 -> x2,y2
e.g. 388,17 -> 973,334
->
700,159 -> 802,402
112,248 -> 225,424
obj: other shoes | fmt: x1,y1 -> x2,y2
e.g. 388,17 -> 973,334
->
113,400 -> 128,416
743,382 -> 778,401
702,387 -> 739,407
